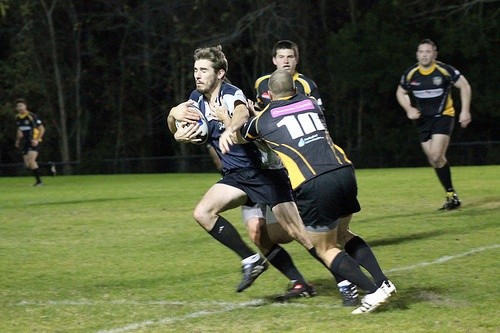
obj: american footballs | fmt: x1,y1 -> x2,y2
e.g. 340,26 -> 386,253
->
175,107 -> 209,144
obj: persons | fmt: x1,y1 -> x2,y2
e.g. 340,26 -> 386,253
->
167,45 -> 358,306
396,40 -> 471,211
207,70 -> 397,315
14,99 -> 46,187
242,39 -> 322,302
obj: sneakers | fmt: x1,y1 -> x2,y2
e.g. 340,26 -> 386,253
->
351,287 -> 389,314
49,161 -> 57,178
438,191 -> 461,211
339,282 -> 359,306
33,181 -> 42,187
383,277 -> 398,297
236,252 -> 271,293
272,279 -> 318,302
